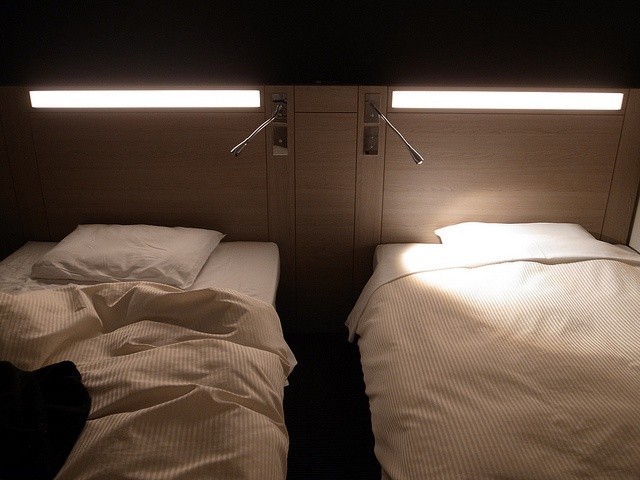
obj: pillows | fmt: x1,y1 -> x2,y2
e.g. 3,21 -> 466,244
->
30,225 -> 227,291
435,222 -> 598,264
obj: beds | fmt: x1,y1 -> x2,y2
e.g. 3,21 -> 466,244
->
346,241 -> 639,480
1,240 -> 298,480
